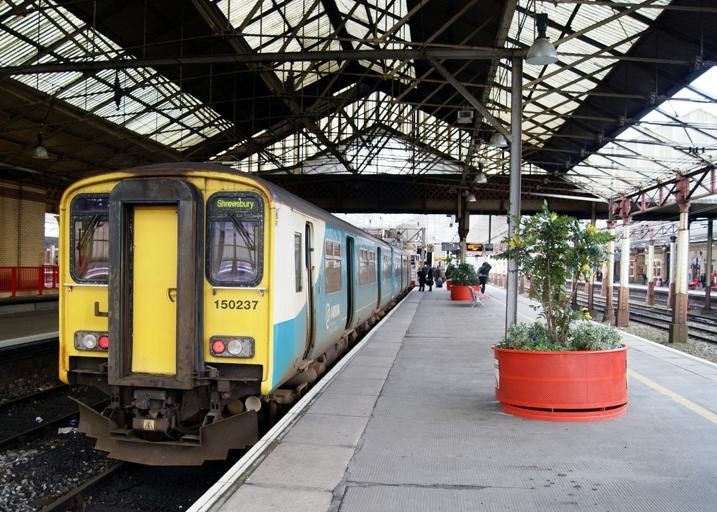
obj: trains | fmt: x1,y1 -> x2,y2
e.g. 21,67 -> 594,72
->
57,161 -> 412,468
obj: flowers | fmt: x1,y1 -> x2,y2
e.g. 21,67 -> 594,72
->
494,199 -> 625,352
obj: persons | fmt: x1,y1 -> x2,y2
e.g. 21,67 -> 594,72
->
701,273 -> 706,288
595,268 -> 602,281
477,262 -> 490,293
417,264 -> 446,292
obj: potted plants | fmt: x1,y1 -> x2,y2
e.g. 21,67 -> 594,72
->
445,261 -> 482,301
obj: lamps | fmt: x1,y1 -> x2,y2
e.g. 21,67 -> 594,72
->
525,1 -> 559,66
465,113 -> 488,202
488,57 -> 509,148
